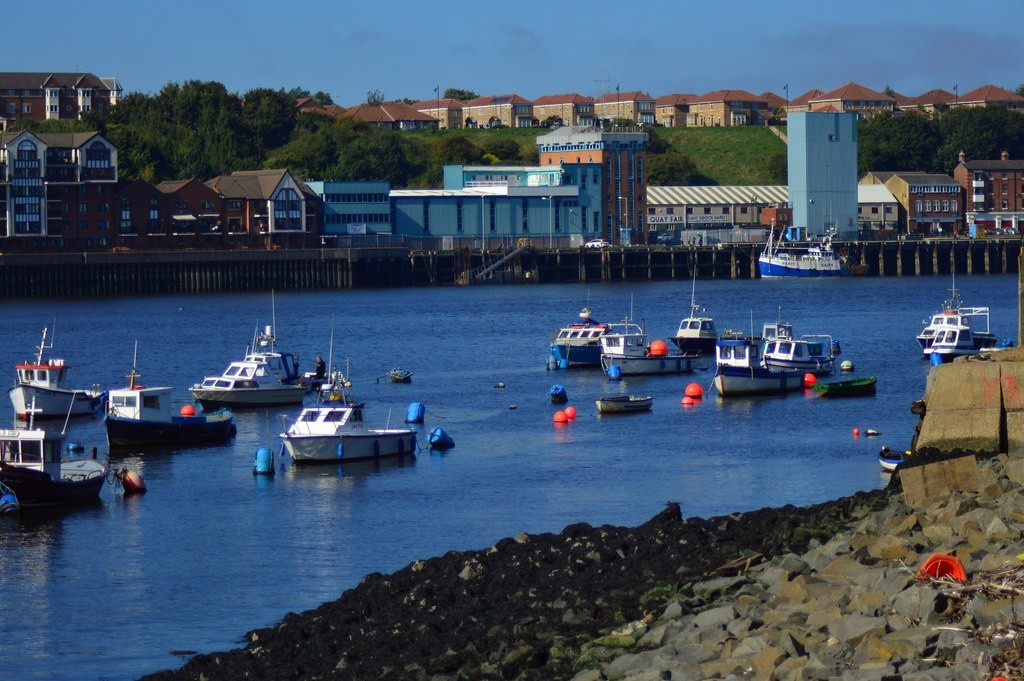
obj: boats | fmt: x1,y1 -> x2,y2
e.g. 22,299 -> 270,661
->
598,292 -> 705,378
878,446 -> 911,472
810,375 -> 877,398
548,289 -> 635,369
0,388 -> 108,509
595,394 -> 654,414
761,303 -> 843,376
299,311 -> 337,394
385,366 -> 413,383
923,299 -> 1004,362
8,315 -> 107,421
187,288 -> 311,408
279,313 -> 419,464
666,263 -> 764,354
757,218 -> 854,278
101,340 -> 236,449
712,309 -> 805,396
915,261 -> 998,349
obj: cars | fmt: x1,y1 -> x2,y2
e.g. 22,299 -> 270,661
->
585,238 -> 609,250
985,226 -> 1020,237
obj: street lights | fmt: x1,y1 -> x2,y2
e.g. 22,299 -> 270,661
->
541,196 -> 553,250
618,196 -> 628,244
481,195 -> 487,251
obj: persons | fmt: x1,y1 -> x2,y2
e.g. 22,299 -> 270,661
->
310,356 -> 326,379
